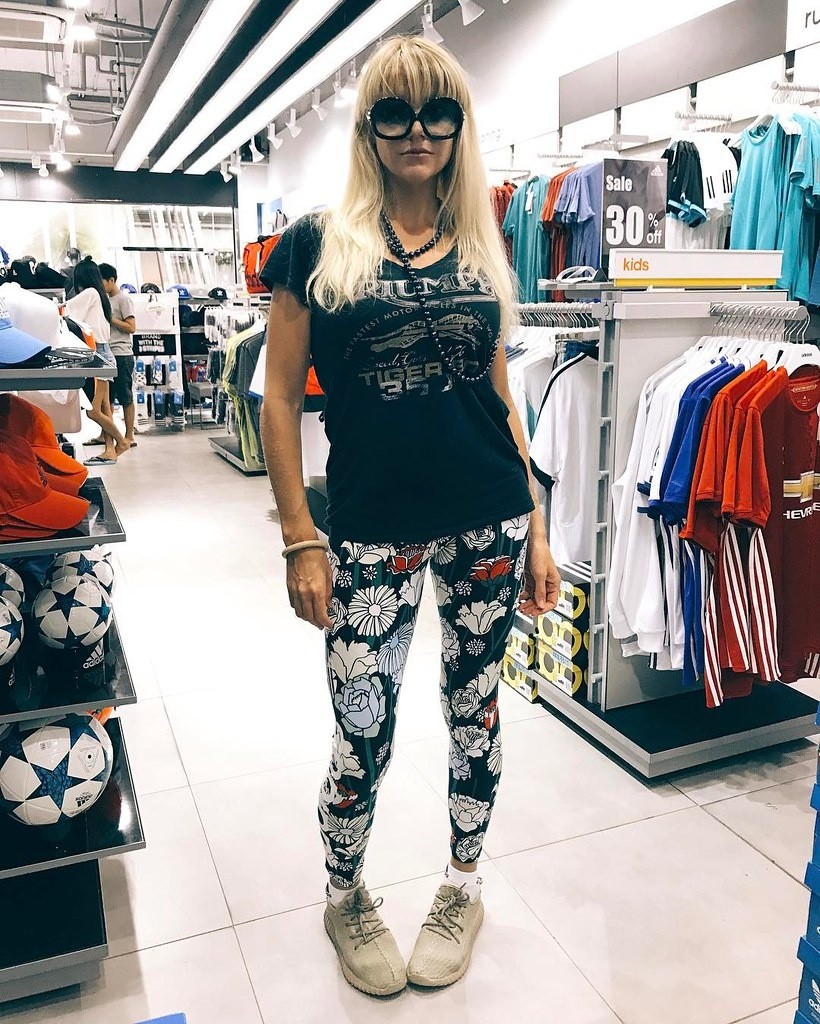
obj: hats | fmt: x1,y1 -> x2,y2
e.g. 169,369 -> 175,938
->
0,281 -> 97,364
0,393 -> 91,541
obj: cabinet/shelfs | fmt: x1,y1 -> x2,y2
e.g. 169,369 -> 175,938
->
0,342 -> 148,1018
178,298 -> 226,409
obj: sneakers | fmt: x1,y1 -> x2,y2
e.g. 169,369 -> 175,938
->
319,868 -> 483,997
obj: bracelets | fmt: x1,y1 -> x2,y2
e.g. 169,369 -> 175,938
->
281,539 -> 330,558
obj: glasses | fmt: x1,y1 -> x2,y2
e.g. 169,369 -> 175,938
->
363,95 -> 469,139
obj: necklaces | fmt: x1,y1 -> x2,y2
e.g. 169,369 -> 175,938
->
376,196 -> 502,381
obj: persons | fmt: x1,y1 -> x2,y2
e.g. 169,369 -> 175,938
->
64,260 -> 137,466
258,36 -> 561,996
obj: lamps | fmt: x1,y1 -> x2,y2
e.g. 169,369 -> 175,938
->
267,123 -> 283,150
30,156 -> 50,179
220,161 -> 233,183
46,85 -> 71,101
229,153 -> 246,176
285,108 -> 302,139
310,88 -> 329,122
64,114 -> 81,137
458,0 -> 485,27
249,136 -> 264,164
331,69 -> 348,109
339,58 -> 357,100
420,0 -> 444,44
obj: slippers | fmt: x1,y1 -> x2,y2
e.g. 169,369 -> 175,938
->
82,438 -> 106,446
83,456 -> 118,465
130,442 -> 137,447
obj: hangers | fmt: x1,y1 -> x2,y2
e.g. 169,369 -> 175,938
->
666,112 -> 732,149
695,303 -> 819,377
728,81 -> 820,147
242,306 -> 269,333
506,301 -> 601,351
487,137 -> 618,186
